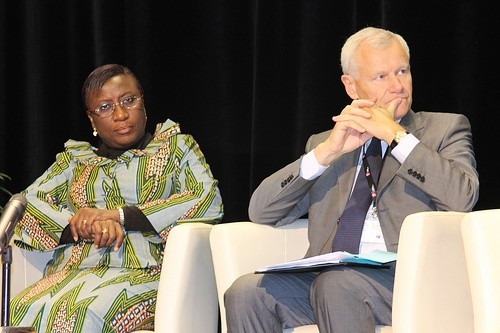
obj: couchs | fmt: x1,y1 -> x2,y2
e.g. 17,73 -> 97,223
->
9,209 -> 500,333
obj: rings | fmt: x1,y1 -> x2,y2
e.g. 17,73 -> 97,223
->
82,219 -> 87,225
101,228 -> 109,234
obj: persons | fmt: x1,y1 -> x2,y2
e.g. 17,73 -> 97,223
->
224,27 -> 480,333
8,64 -> 224,333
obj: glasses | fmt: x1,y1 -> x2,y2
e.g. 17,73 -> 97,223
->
86,94 -> 143,118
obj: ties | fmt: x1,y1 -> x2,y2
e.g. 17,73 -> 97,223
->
332,136 -> 382,254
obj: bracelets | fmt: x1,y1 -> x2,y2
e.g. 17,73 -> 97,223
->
115,205 -> 125,226
390,130 -> 409,150
